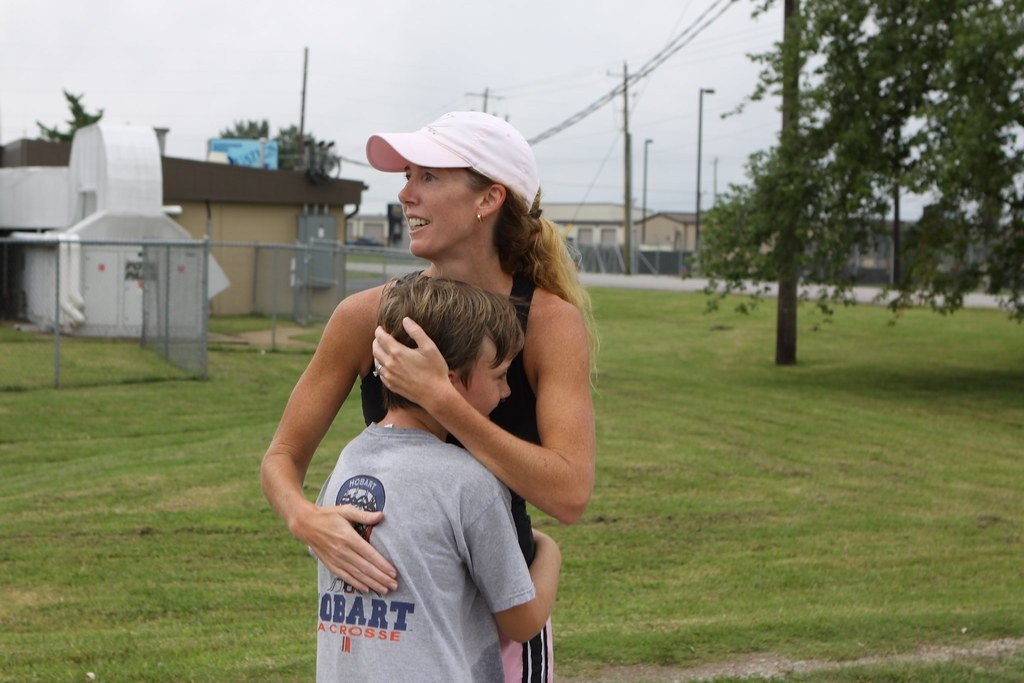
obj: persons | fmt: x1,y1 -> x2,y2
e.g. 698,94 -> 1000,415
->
263,110 -> 595,683
311,275 -> 562,683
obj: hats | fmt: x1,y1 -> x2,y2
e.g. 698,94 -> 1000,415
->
367,111 -> 538,217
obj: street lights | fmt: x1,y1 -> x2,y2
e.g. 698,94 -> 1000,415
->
641,137 -> 654,248
696,88 -> 717,253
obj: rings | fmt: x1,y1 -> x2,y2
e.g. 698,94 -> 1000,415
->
372,364 -> 382,377
